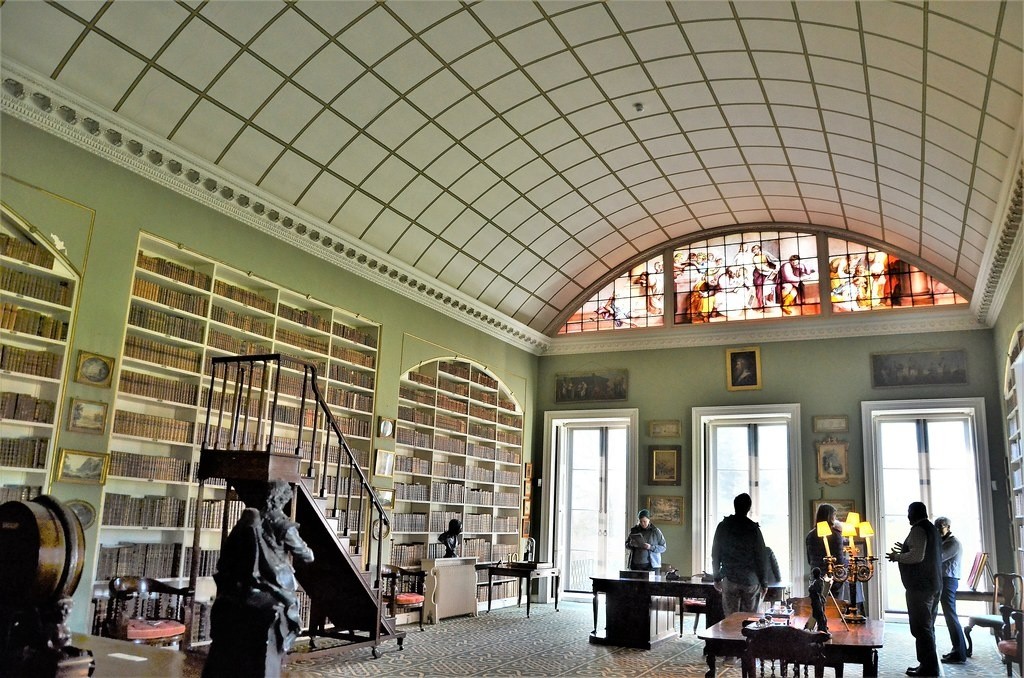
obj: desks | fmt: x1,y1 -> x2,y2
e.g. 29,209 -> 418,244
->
589,576 -> 792,650
486,564 -> 561,618
955,591 -> 1019,635
697,612 -> 885,678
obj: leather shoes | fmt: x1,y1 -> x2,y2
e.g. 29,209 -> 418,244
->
940,654 -> 962,664
905,666 -> 920,677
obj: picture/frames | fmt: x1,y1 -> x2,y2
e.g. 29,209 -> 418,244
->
649,420 -> 682,438
372,519 -> 390,541
523,499 -> 535,516
377,416 -> 397,440
813,416 -> 849,433
815,436 -> 850,487
647,445 -> 681,486
869,348 -> 969,389
371,488 -> 397,510
647,495 -> 685,525
555,368 -> 629,404
66,397 -> 109,435
525,463 -> 536,478
522,519 -> 533,538
524,480 -> 535,496
63,500 -> 96,532
725,346 -> 763,391
812,499 -> 856,528
54,447 -> 111,486
73,350 -> 115,389
374,449 -> 396,479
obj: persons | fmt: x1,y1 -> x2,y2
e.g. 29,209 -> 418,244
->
439,519 -> 461,558
712,493 -> 767,667
934,517 -> 967,664
885,503 -> 944,677
732,359 -> 756,387
213,478 -> 315,652
808,568 -> 829,633
807,504 -> 845,599
625,509 -> 667,575
764,546 -> 781,583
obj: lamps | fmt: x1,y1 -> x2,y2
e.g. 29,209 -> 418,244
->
817,512 -> 874,557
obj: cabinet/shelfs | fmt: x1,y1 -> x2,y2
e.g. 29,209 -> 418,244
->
1003,320 -> 1024,612
85,228 -> 383,652
420,557 -> 478,624
0,200 -> 84,504
384,356 -> 524,625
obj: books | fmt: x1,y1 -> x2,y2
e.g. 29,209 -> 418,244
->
629,533 -> 645,548
97,252 -> 378,646
391,362 -> 524,611
0,231 -> 75,507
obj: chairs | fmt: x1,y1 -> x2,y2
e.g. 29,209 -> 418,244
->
101,575 -> 195,652
964,573 -> 1024,658
680,574 -> 712,637
998,604 -> 1024,676
741,622 -> 833,678
366,564 -> 428,631
